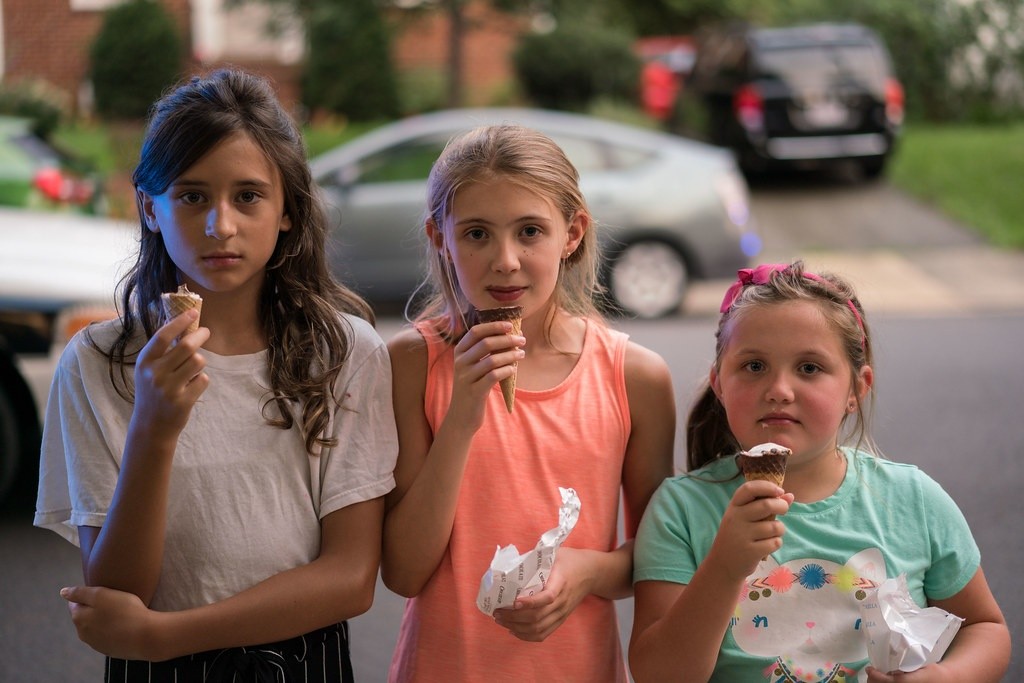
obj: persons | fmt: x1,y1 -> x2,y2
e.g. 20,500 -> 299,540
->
629,262 -> 1011,683
379,125 -> 675,683
32,69 -> 398,683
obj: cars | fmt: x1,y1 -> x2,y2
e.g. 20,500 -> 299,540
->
0,206 -> 146,523
309,106 -> 760,322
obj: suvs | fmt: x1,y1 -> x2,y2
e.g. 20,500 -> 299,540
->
0,113 -> 114,216
663,21 -> 906,185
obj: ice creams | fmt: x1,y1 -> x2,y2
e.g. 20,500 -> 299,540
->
739,444 -> 792,562
162,283 -> 203,344
476,305 -> 524,414
